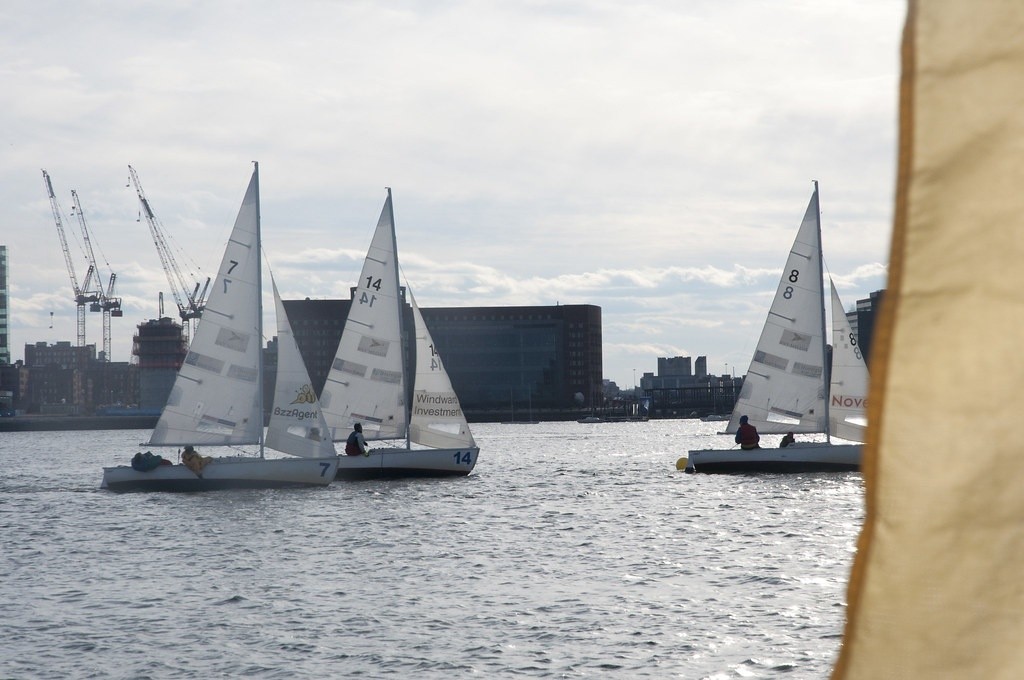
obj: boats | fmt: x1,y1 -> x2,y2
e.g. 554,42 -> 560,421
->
700,415 -> 724,422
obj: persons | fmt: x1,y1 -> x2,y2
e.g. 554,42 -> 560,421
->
345,422 -> 368,456
309,428 -> 321,442
132,451 -> 171,472
181,446 -> 213,479
779,432 -> 795,448
735,416 -> 760,450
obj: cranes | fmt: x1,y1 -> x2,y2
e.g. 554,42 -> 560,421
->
126,165 -> 211,366
71,189 -> 122,403
42,169 -> 101,415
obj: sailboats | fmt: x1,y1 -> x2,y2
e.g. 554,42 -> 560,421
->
686,181 -> 870,476
102,161 -> 340,493
319,187 -> 480,481
578,397 -> 603,423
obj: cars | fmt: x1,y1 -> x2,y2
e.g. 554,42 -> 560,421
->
0,407 -> 15,417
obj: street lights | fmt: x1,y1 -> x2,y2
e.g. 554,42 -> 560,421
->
725,363 -> 727,374
633,369 -> 636,386
